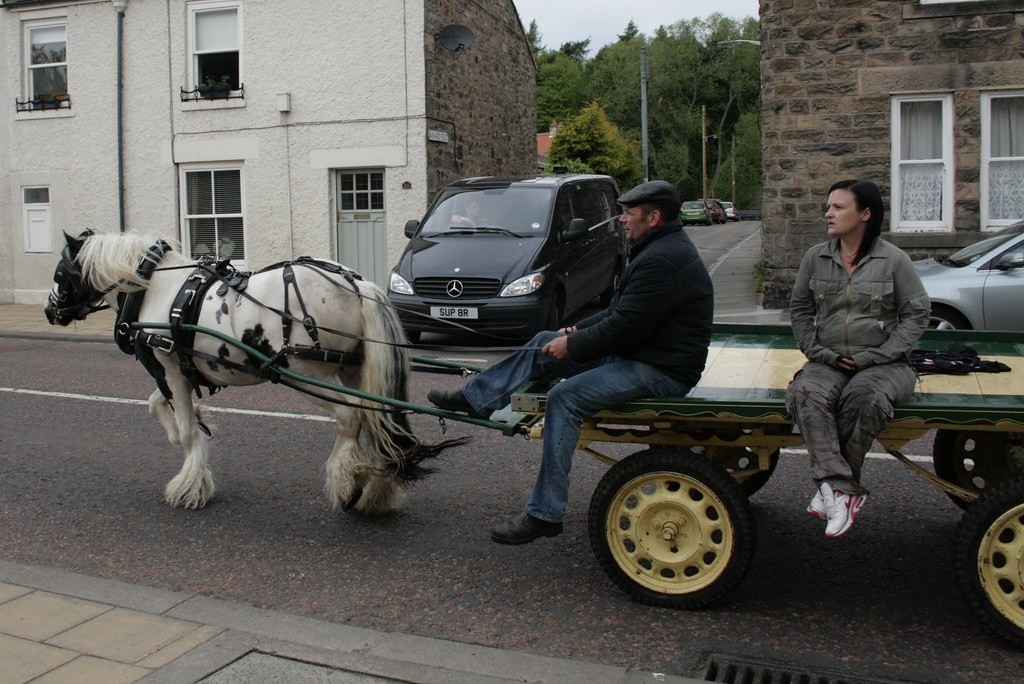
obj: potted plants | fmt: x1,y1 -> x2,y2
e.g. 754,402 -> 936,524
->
198,74 -> 230,100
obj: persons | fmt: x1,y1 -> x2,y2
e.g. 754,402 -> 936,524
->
784,179 -> 932,537
427,180 -> 715,544
450,199 -> 490,228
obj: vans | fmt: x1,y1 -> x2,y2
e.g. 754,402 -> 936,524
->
384,173 -> 631,349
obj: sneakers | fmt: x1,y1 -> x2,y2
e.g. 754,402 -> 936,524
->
806,488 -> 828,520
820,482 -> 868,536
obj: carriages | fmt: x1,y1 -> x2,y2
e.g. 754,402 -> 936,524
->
46,230 -> 1024,653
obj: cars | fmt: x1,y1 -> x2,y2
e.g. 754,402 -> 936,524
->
680,201 -> 711,226
699,199 -> 727,225
909,220 -> 1024,333
720,202 -> 740,222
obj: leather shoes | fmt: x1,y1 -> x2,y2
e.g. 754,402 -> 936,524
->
491,512 -> 565,545
427,387 -> 487,420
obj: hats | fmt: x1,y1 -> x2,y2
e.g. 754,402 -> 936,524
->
615,180 -> 678,206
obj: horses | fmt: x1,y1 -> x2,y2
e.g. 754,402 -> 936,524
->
44,228 -> 475,516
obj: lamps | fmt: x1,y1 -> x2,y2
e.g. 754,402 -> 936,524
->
192,243 -> 211,260
